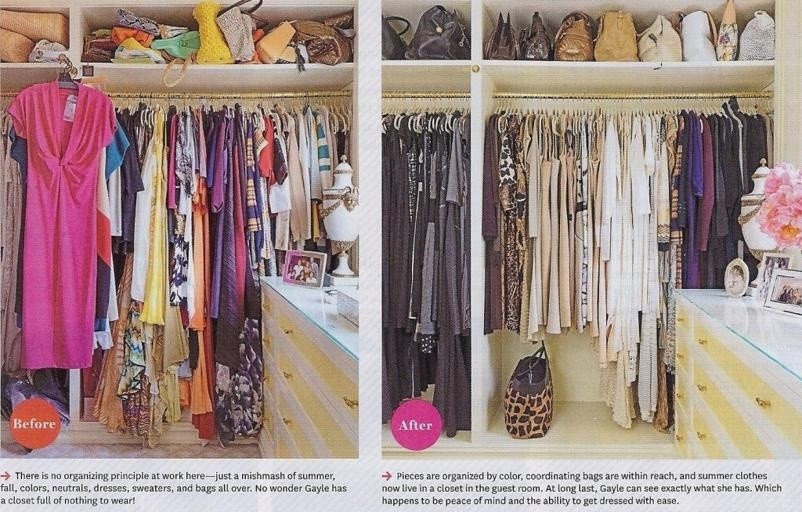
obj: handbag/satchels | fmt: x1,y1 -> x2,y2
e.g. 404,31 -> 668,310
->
0,6 -> 71,63
483,1 -> 776,63
404,3 -> 472,60
80,0 -> 357,89
381,11 -> 410,60
502,339 -> 555,442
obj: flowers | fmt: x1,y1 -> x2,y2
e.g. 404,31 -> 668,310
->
759,159 -> 802,250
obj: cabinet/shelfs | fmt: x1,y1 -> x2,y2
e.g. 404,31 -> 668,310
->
260,270 -> 358,456
672,288 -> 802,460
1,0 -> 360,457
382,2 -> 802,458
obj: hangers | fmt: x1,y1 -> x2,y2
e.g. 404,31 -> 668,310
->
54,54 -> 79,91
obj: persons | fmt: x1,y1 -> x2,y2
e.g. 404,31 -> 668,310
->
779,283 -> 802,306
762,258 -> 773,302
779,258 -> 787,268
729,268 -> 745,291
286,256 -> 320,283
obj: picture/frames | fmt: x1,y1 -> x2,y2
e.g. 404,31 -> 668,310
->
723,250 -> 802,317
281,247 -> 327,292
